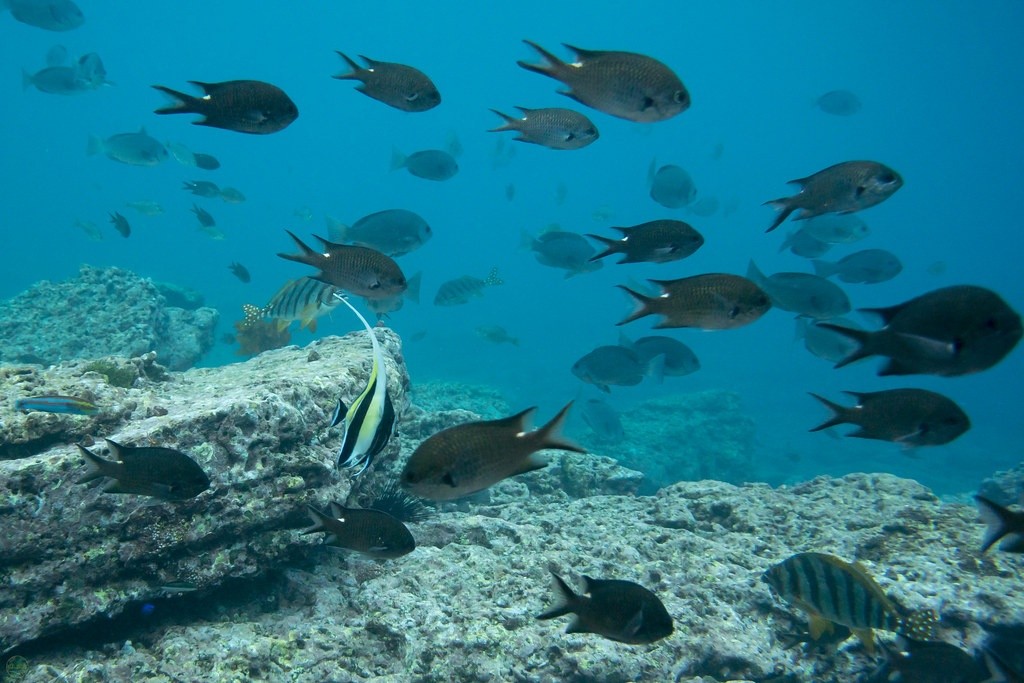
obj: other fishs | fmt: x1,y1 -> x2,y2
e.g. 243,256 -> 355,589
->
0,0 -> 1024,683
